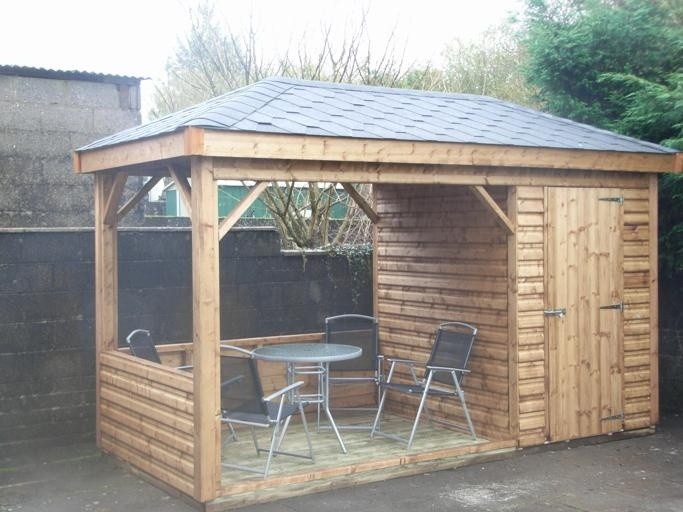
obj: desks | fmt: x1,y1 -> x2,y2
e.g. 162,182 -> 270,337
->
252,343 -> 364,456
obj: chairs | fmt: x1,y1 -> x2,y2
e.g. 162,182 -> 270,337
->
316,314 -> 386,432
126,328 -> 196,370
366,320 -> 477,449
221,341 -> 315,481
